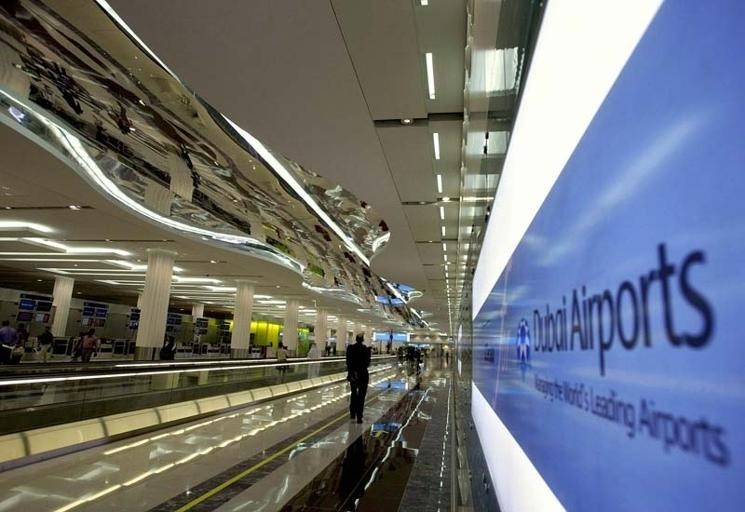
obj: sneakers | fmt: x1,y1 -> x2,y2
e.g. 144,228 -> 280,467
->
350,416 -> 363,424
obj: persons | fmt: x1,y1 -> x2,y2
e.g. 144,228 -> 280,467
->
346,331 -> 371,425
0,319 -> 98,364
397,344 -> 422,363
277,341 -> 288,381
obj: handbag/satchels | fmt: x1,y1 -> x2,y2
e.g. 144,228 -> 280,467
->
12,347 -> 25,356
